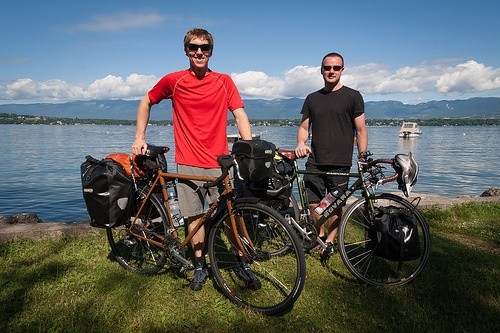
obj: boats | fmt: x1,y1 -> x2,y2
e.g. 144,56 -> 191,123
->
398,121 -> 422,139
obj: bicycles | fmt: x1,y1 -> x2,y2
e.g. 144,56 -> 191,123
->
106,137 -> 307,316
235,152 -> 431,288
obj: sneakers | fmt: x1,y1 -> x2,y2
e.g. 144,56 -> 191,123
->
233,264 -> 262,290
190,266 -> 209,291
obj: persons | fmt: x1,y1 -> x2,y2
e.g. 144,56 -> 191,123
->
295,51 -> 369,259
132,27 -> 262,292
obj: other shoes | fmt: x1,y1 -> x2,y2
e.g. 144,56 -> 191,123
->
320,242 -> 334,259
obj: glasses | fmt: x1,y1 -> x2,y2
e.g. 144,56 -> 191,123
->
323,66 -> 343,72
187,43 -> 211,52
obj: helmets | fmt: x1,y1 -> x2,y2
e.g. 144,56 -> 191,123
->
393,151 -> 420,197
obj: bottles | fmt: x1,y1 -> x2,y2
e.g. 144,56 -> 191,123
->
313,190 -> 343,216
167,187 -> 185,228
